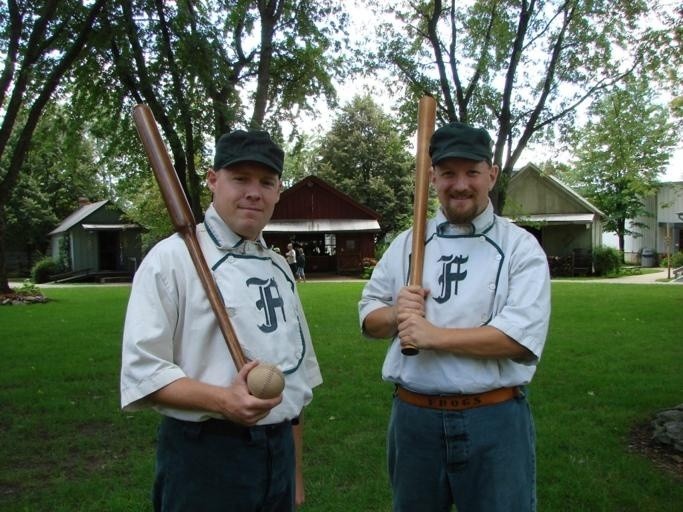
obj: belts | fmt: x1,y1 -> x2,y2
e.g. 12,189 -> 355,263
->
395,386 -> 517,410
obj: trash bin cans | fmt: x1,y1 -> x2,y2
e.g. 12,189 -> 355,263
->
641,249 -> 654,267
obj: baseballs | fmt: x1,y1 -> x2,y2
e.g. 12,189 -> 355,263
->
246,363 -> 285,399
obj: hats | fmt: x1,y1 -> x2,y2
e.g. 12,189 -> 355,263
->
428,122 -> 494,168
213,129 -> 284,179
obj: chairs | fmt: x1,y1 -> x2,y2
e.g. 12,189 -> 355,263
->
571,248 -> 586,275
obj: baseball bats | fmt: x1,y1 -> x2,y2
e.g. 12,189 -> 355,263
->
133,104 -> 245,372
402,96 -> 436,356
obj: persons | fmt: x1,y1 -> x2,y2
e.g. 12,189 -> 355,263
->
294,248 -> 306,282
357,121 -> 554,512
273,247 -> 281,256
284,243 -> 296,272
118,132 -> 324,511
